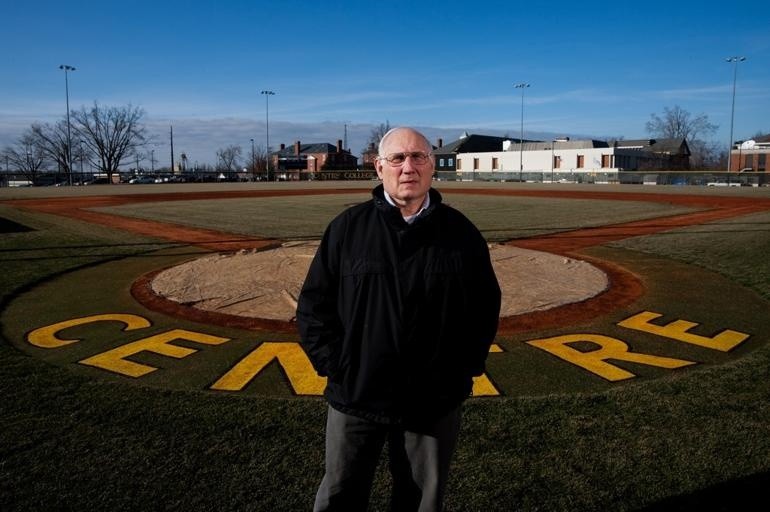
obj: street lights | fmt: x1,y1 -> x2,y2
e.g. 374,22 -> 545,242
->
261,91 -> 275,181
57,65 -> 77,186
514,84 -> 530,182
726,55 -> 745,172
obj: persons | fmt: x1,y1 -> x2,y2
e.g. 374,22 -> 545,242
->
294,126 -> 501,512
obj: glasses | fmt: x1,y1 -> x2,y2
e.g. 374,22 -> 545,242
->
375,150 -> 432,167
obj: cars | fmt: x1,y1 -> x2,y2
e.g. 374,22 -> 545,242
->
129,175 -> 155,184
706,178 -> 743,187
